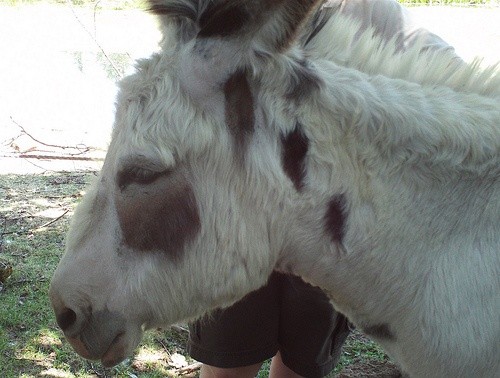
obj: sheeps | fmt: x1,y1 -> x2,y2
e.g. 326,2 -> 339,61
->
47,0 -> 499,378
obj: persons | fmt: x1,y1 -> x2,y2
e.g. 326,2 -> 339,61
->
188,0 -> 407,378
